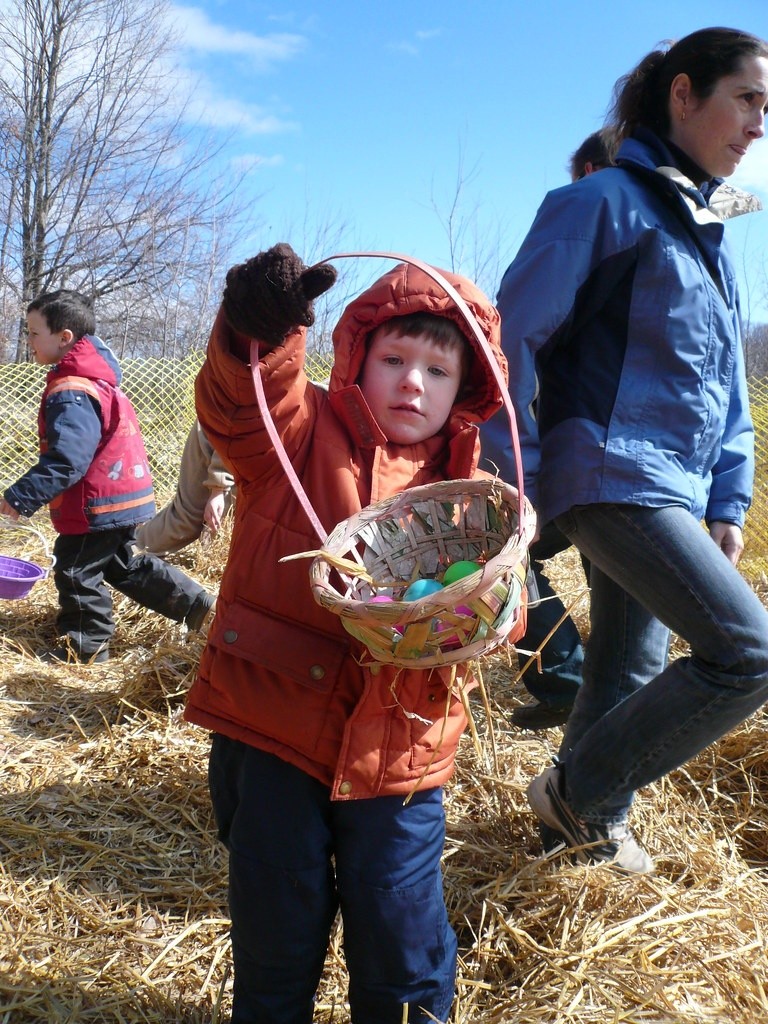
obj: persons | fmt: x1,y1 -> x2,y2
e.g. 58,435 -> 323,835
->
196,243 -> 526,1024
2,27 -> 768,873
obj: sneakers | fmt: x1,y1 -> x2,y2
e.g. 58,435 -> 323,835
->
525,753 -> 658,876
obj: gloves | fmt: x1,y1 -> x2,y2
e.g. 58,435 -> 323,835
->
221,240 -> 338,342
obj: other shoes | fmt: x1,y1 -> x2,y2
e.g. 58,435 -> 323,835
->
185,590 -> 218,638
34,643 -> 110,666
509,700 -> 579,731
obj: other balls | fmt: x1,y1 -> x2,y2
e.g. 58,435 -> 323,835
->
358,553 -> 499,652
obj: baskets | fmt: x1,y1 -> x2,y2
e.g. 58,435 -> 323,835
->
249,249 -> 537,668
